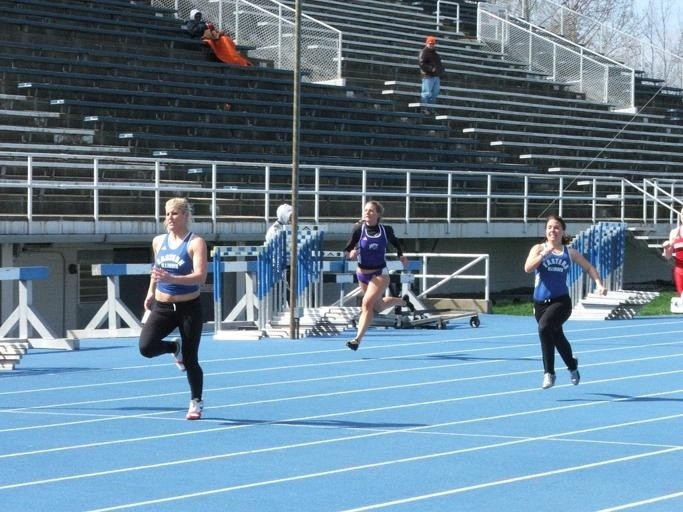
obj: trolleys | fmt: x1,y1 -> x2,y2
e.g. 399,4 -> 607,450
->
385,274 -> 480,330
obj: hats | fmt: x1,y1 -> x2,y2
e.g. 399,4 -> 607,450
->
427,38 -> 434,44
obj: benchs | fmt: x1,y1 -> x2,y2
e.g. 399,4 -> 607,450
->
0,0 -> 682,213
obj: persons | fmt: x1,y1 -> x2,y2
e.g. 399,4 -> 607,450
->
418,34 -> 446,115
523,213 -> 609,391
343,200 -> 416,351
186,7 -> 209,39
137,196 -> 208,420
262,200 -> 293,310
661,207 -> 682,296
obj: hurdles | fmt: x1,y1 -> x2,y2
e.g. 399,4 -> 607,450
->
533,221 -> 660,320
0,224 -> 422,371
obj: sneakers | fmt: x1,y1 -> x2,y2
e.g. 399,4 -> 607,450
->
172,339 -> 186,371
569,358 -> 580,385
402,295 -> 414,312
186,399 -> 203,420
542,372 -> 555,388
424,110 -> 438,115
345,339 -> 359,351
